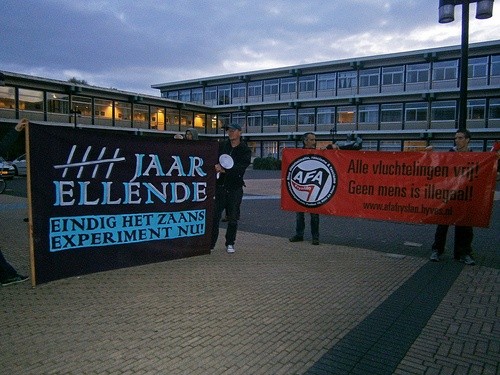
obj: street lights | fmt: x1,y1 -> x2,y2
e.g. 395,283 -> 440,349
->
438,0 -> 494,130
220,123 -> 229,141
70,106 -> 82,128
329,127 -> 337,144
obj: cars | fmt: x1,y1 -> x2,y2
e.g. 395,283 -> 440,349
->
0,156 -> 16,179
10,153 -> 27,177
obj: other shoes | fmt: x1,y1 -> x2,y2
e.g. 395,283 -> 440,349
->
1,274 -> 29,286
289,235 -> 303,242
226,245 -> 235,252
459,255 -> 475,265
313,239 -> 319,245
430,251 -> 440,262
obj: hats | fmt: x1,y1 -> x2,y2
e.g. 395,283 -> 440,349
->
225,124 -> 241,131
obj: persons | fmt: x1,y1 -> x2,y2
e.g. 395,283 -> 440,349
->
185,128 -> 198,140
0,118 -> 30,286
424,128 -> 477,266
281,133 -> 340,246
213,123 -> 251,253
